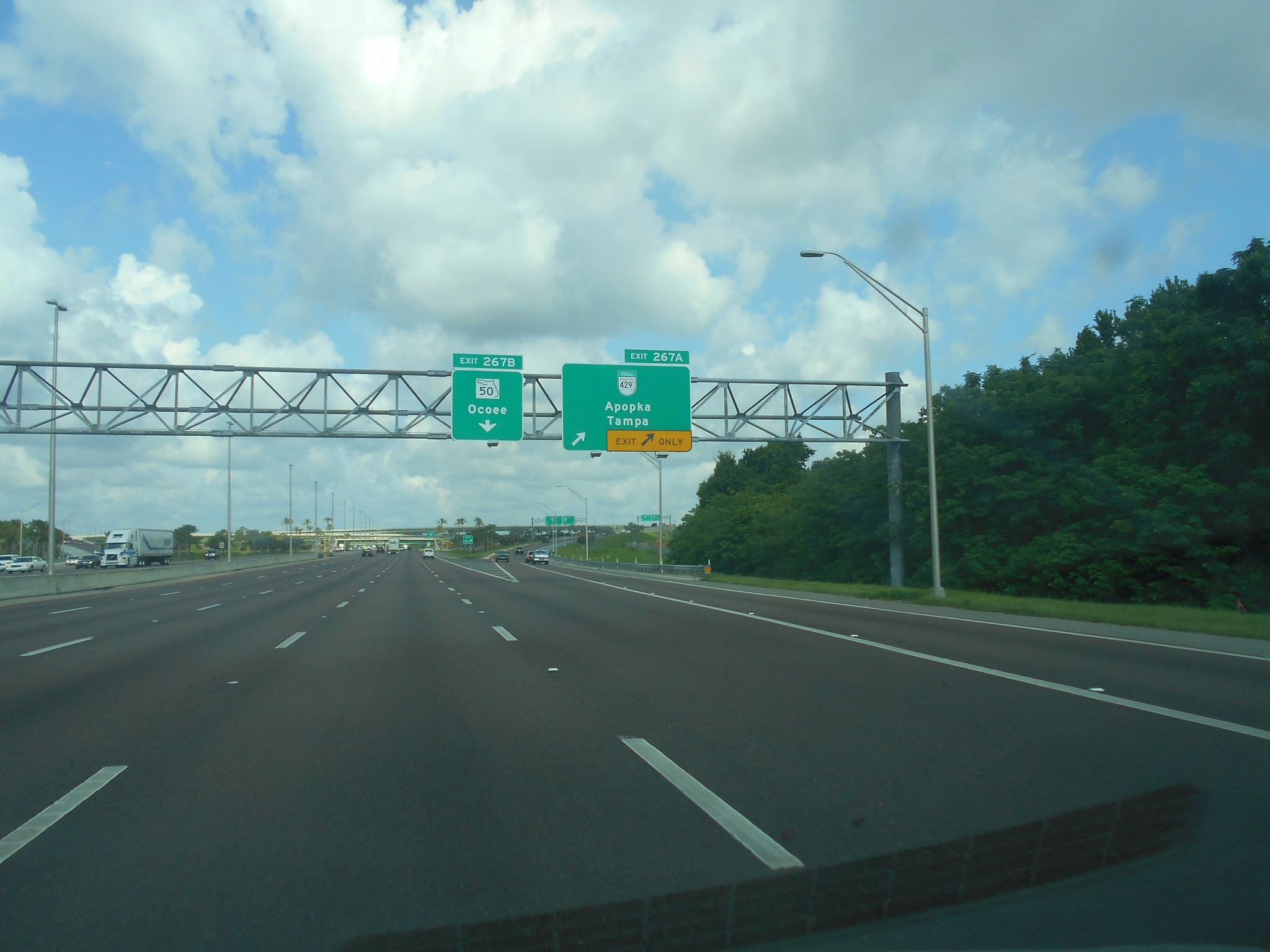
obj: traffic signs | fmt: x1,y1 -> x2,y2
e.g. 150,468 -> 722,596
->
463,535 -> 473,544
452,370 -> 523,440
562,363 -> 692,452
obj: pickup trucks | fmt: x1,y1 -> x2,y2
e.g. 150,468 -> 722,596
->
533,550 -> 549,565
423,547 -> 435,559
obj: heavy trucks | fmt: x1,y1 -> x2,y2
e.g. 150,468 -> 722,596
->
100,527 -> 174,567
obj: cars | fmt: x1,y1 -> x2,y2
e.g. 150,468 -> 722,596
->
66,557 -> 81,566
0,554 -> 19,572
75,555 -> 102,569
6,557 -> 48,573
400,546 -> 412,551
64,540 -> 104,557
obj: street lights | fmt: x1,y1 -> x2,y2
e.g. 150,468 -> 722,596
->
534,485 -> 589,561
47,299 -> 68,574
799,249 -> 947,596
288,464 -> 389,556
227,421 -> 234,561
19,500 -> 49,557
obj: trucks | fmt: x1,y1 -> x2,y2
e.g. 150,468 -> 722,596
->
388,536 -> 400,554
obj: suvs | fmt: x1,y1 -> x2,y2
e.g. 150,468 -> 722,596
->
204,549 -> 220,560
495,550 -> 510,562
515,547 -> 524,555
525,551 -> 534,563
